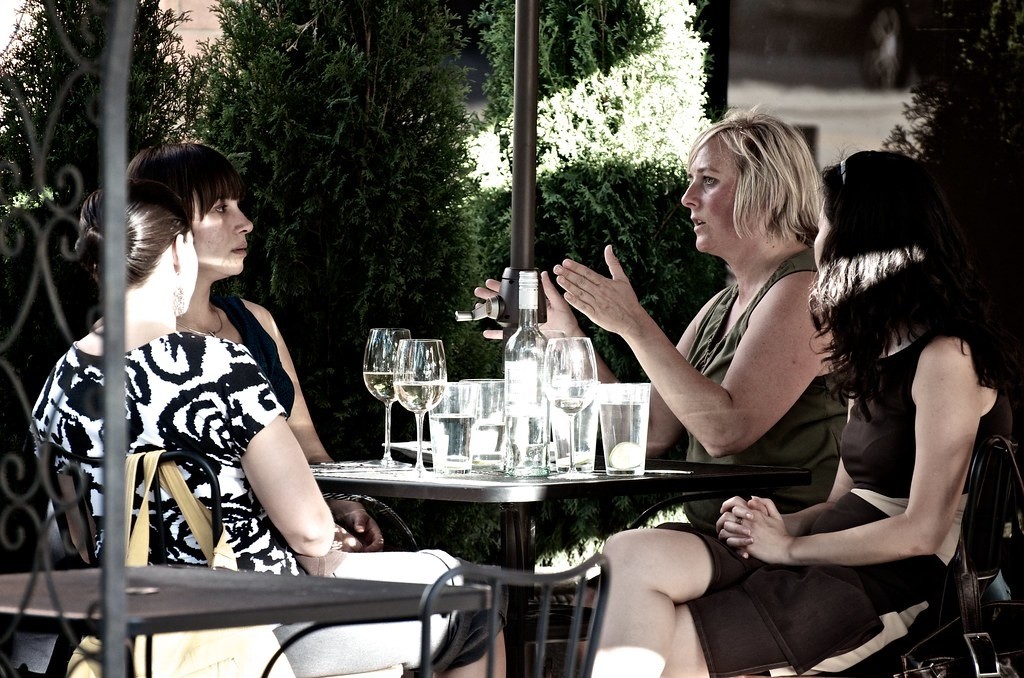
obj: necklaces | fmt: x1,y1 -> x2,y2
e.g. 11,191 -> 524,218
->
176,302 -> 222,336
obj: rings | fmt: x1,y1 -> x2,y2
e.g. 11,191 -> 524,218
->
735,517 -> 742,524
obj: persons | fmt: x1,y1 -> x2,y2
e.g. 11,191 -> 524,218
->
31,179 -> 506,676
474,110 -> 847,525
127,143 -> 385,553
590,151 -> 1024,678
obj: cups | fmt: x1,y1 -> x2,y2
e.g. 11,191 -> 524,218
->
428,381 -> 480,475
544,381 -> 597,472
597,380 -> 653,477
463,378 -> 507,472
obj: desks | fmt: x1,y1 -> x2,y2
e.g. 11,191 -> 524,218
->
310,459 -> 812,678
0,566 -> 490,678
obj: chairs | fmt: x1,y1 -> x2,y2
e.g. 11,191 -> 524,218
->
941,437 -> 1024,627
420,553 -> 613,678
37,443 -> 222,568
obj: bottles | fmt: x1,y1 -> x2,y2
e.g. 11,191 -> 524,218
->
502,271 -> 554,477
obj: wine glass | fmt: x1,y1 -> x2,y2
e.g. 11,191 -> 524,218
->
540,336 -> 598,478
363,327 -> 414,470
394,338 -> 448,475
